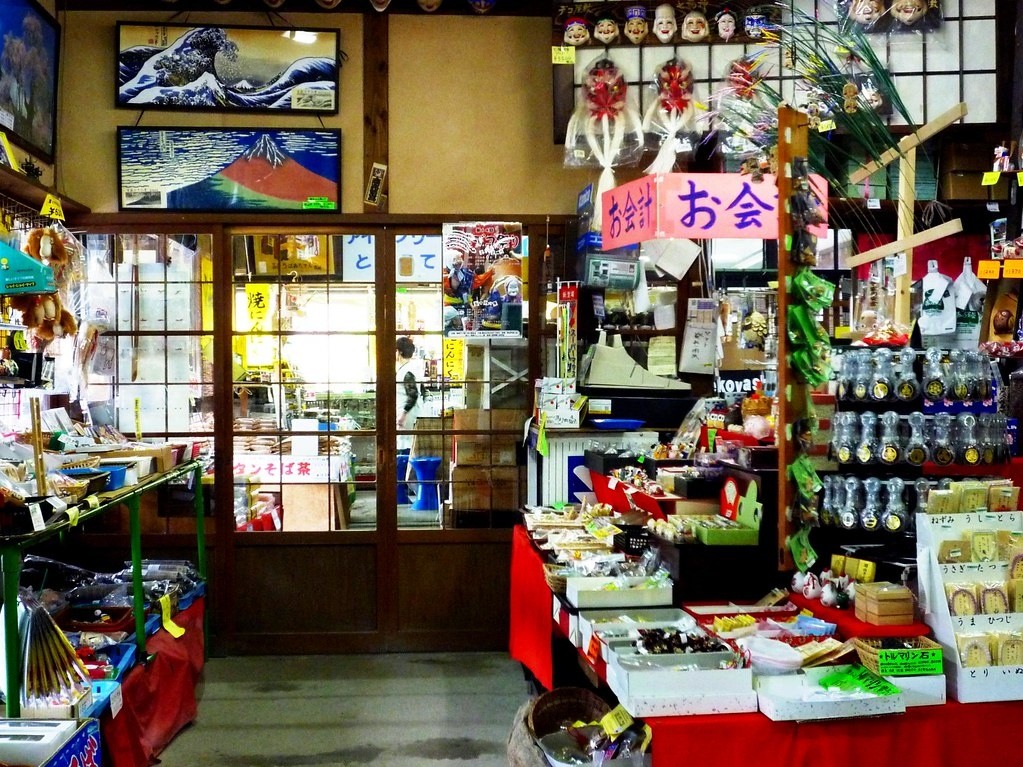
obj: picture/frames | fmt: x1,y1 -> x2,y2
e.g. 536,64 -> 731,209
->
0,0 -> 61,165
116,21 -> 341,114
116,126 -> 343,214
248,235 -> 343,279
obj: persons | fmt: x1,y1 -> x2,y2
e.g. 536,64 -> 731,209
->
396,337 -> 426,496
444,305 -> 461,337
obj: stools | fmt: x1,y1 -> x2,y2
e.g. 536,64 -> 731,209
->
396,454 -> 413,505
409,456 -> 443,510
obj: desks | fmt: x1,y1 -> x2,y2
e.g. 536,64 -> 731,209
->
508,525 -> 579,696
405,417 -> 454,494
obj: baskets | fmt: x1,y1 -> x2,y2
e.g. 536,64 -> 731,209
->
53,605 -> 137,642
53,477 -> 90,501
527,685 -> 612,742
62,453 -> 102,471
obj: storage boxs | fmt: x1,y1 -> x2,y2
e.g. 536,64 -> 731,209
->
89,447 -> 172,473
102,457 -> 153,478
578,601 -> 906,721
584,448 -> 763,546
442,409 -> 529,528
738,446 -> 778,469
577,509 -> 1023,767
20,687 -> 93,718
718,131 -> 1009,200
281,483 -> 347,531
0,720 -> 77,766
535,376 -> 581,430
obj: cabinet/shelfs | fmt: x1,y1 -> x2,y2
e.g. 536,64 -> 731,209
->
0,454 -> 205,767
